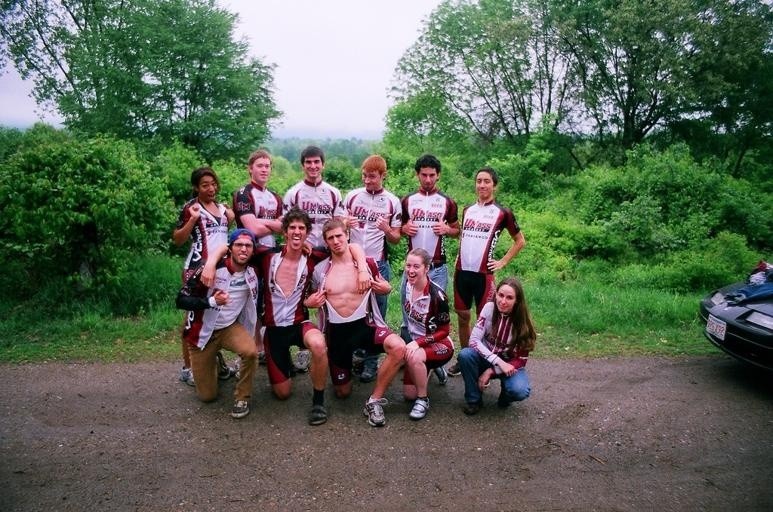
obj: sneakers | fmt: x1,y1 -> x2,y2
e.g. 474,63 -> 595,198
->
408,396 -> 430,420
178,364 -> 197,387
231,399 -> 252,419
433,366 -> 449,386
445,362 -> 464,377
362,398 -> 387,427
308,404 -> 329,426
257,348 -> 267,364
463,395 -> 484,417
295,350 -> 311,373
496,380 -> 513,409
358,356 -> 379,384
216,351 -> 241,380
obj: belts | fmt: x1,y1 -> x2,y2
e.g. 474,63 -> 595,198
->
430,261 -> 444,270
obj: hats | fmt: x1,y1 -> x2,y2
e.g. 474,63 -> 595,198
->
227,227 -> 256,248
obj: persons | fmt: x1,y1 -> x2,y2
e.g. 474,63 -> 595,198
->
447,167 -> 525,377
174,228 -> 262,420
457,278 -> 536,416
199,208 -> 372,427
172,166 -> 236,387
343,154 -> 402,383
304,219 -> 407,427
233,149 -> 283,365
396,248 -> 454,419
400,154 -> 461,344
281,146 -> 342,374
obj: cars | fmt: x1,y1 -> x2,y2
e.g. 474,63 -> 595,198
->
691,267 -> 772,372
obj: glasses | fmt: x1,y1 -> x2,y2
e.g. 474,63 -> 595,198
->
231,240 -> 254,249
